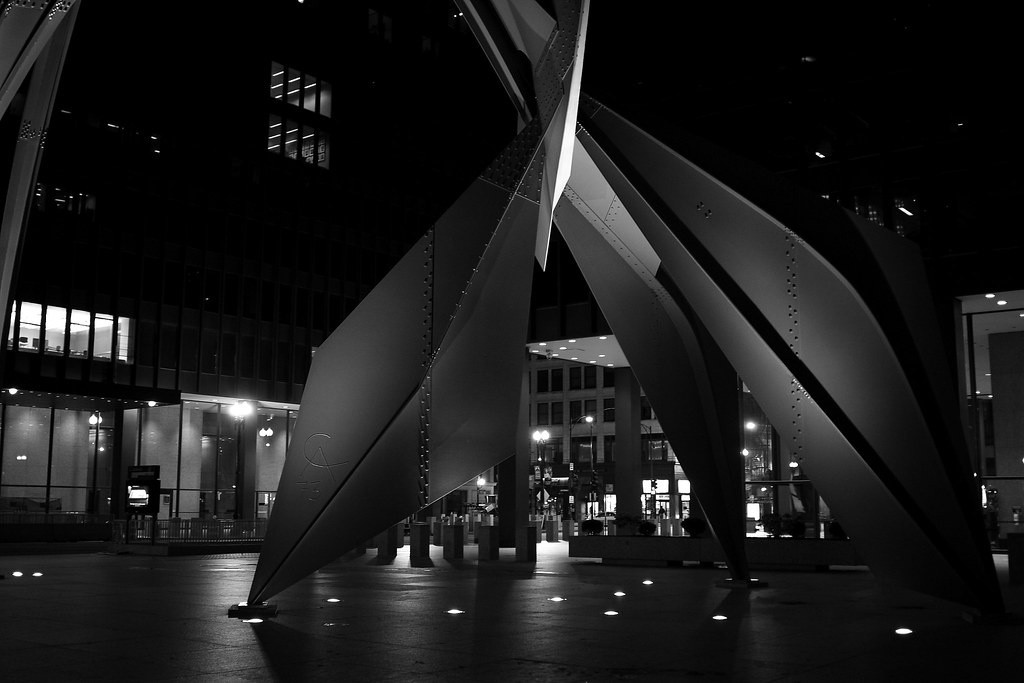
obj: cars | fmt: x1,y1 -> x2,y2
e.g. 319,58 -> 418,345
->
592,511 -> 616,523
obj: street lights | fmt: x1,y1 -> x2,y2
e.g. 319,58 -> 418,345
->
476,473 -> 486,509
532,429 -> 550,514
569,415 -> 593,519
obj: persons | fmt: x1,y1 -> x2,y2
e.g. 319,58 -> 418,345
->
658,506 -> 667,520
